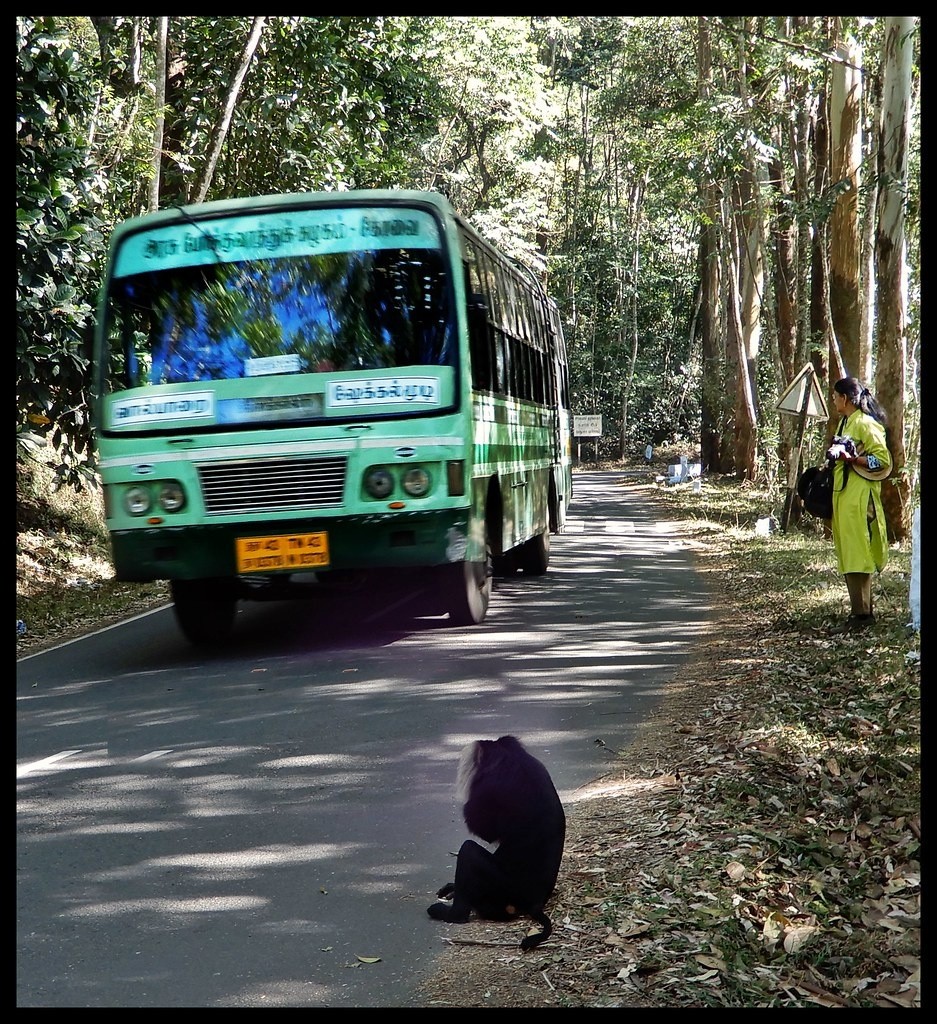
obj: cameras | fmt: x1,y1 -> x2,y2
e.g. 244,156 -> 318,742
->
826,434 -> 857,461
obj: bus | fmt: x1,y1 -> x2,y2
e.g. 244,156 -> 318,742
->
91,189 -> 574,647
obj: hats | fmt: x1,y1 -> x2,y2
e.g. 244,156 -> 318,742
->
850,441 -> 893,481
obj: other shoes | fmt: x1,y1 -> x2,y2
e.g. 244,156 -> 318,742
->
827,612 -> 877,636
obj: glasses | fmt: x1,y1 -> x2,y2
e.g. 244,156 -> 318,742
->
831,394 -> 844,399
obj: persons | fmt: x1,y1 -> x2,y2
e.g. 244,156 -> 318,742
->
823,377 -> 894,626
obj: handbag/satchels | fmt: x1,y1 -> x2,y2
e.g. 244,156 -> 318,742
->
796,464 -> 833,520
